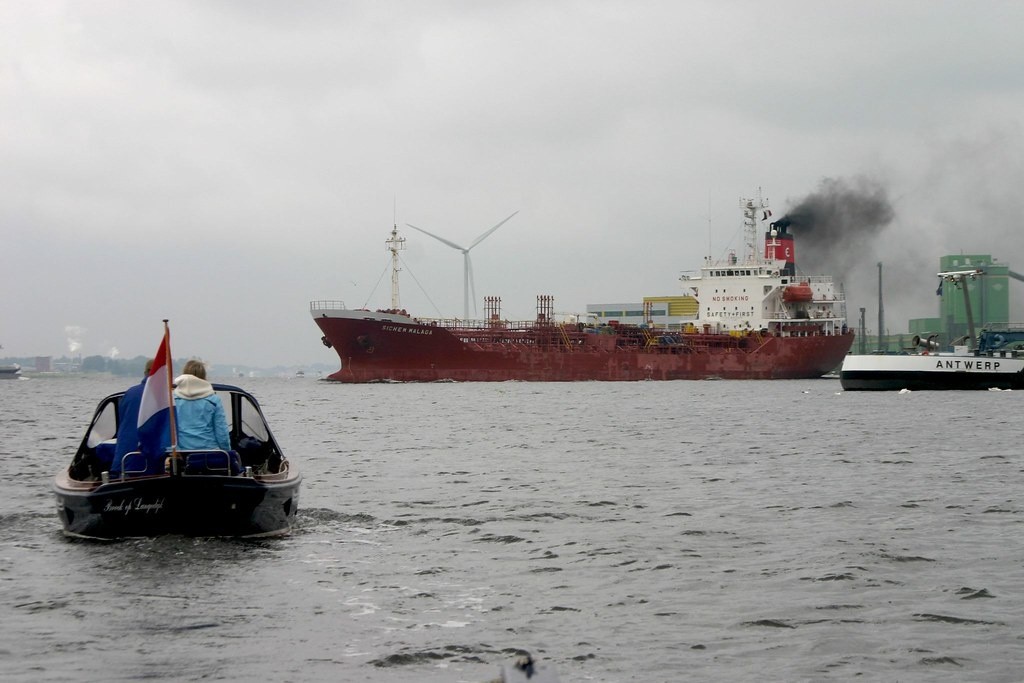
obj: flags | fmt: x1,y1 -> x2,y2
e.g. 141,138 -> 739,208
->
137,327 -> 176,428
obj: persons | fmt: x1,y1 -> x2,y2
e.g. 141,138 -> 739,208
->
173,359 -> 231,450
110,358 -> 171,475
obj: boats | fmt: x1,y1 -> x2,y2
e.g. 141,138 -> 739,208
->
841,269 -> 1024,391
0,363 -> 21,379
50,317 -> 301,544
309,183 -> 856,382
